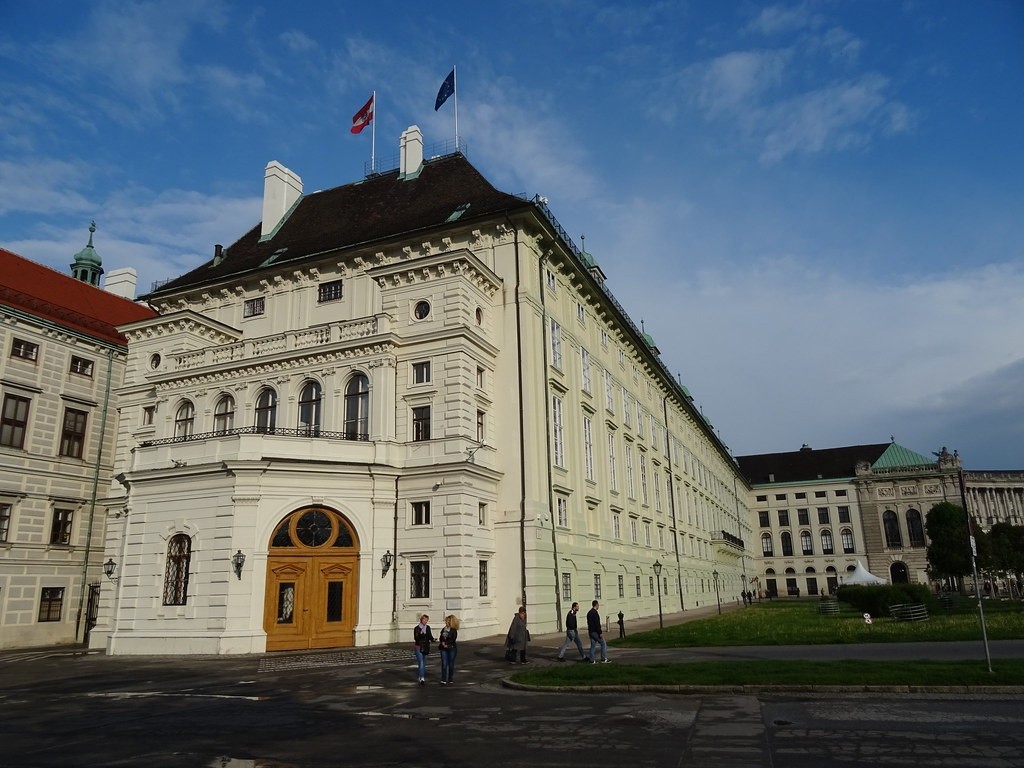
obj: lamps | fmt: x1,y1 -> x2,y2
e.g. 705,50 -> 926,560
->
233,549 -> 246,580
436,481 -> 443,486
537,513 -> 549,522
104,559 -> 119,585
382,550 -> 394,578
115,509 -> 124,515
466,438 -> 487,461
539,196 -> 548,205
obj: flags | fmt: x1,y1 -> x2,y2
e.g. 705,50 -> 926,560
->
351,95 -> 374,134
434,69 -> 454,112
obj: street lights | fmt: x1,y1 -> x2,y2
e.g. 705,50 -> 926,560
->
653,559 -> 665,629
755,576 -> 760,602
740,574 -> 748,607
712,570 -> 721,615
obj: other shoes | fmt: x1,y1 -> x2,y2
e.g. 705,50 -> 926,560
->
418,676 -> 425,688
582,656 -> 590,662
449,682 -> 453,684
440,681 -> 446,685
521,661 -> 530,664
509,662 -> 516,665
557,658 -> 566,662
601,658 -> 612,663
589,660 -> 599,664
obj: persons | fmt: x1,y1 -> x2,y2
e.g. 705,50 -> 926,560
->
586,600 -> 612,664
438,615 -> 459,686
414,614 -> 437,685
821,588 -> 824,596
919,578 -> 1024,597
556,602 -> 590,662
503,607 -> 531,664
741,589 -> 772,608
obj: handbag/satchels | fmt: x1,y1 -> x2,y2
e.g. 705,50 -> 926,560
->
525,630 -> 531,642
420,639 -> 430,655
505,639 -> 517,661
